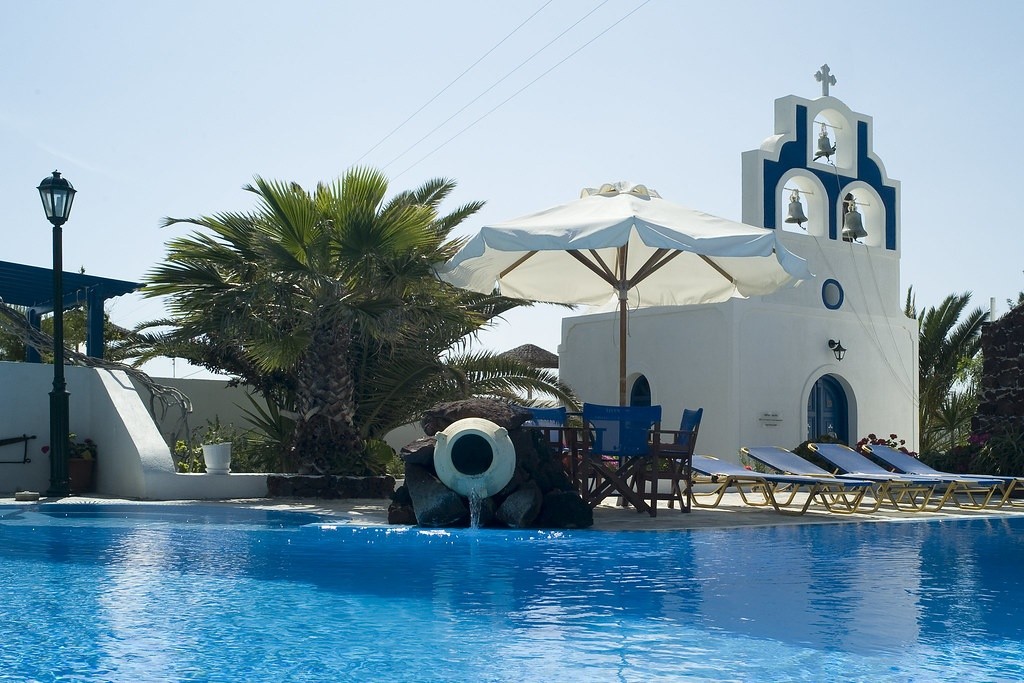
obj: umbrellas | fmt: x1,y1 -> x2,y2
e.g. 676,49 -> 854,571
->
436,180 -> 816,504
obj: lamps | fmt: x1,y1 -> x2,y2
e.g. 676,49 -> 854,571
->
829,340 -> 847,361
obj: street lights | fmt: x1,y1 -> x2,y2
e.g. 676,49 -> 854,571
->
37,170 -> 82,498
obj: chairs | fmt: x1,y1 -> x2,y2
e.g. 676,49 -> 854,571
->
523,402 -> 703,518
666,443 -> 1024,516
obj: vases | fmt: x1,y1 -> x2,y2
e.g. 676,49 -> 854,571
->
203,442 -> 232,474
69,433 -> 97,460
71,460 -> 93,490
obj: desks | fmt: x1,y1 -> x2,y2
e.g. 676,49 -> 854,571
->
523,425 -> 607,505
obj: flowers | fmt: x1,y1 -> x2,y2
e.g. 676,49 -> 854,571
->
203,431 -> 224,444
